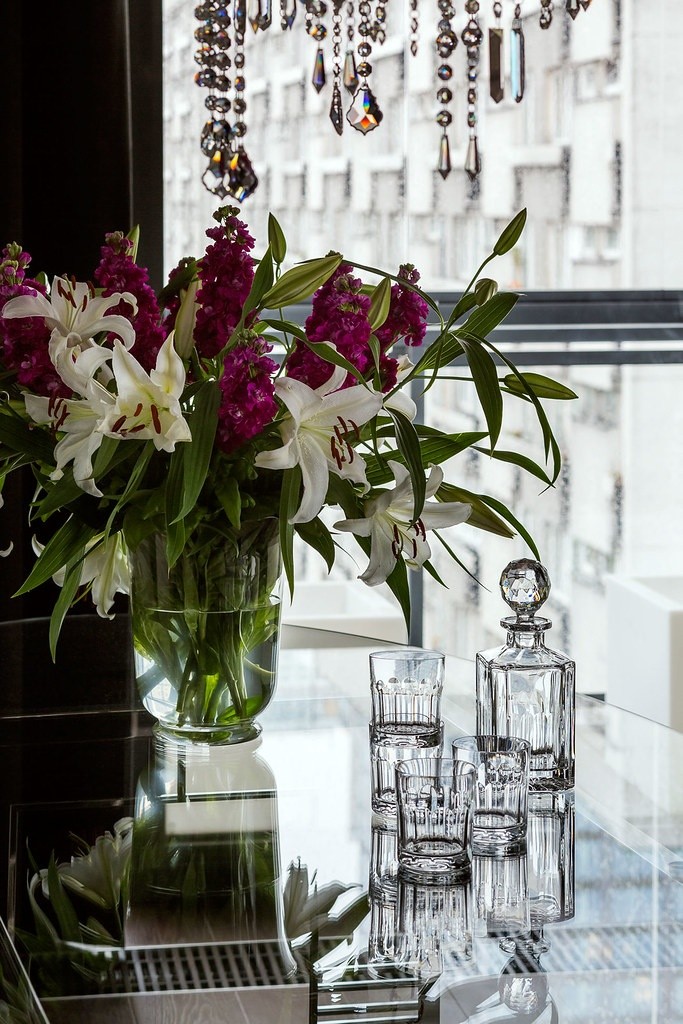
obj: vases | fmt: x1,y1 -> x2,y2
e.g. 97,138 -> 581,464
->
130,514 -> 284,749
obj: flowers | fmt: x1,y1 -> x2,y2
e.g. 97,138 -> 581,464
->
0,204 -> 582,731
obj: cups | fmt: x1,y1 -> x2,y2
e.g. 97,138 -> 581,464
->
451,735 -> 532,845
368,808 -> 529,980
393,757 -> 477,873
367,739 -> 446,814
367,649 -> 446,748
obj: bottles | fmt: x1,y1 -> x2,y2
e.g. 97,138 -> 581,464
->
499,794 -> 576,1013
473,558 -> 577,794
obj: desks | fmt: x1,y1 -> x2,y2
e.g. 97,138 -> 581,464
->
0,615 -> 683,1024
